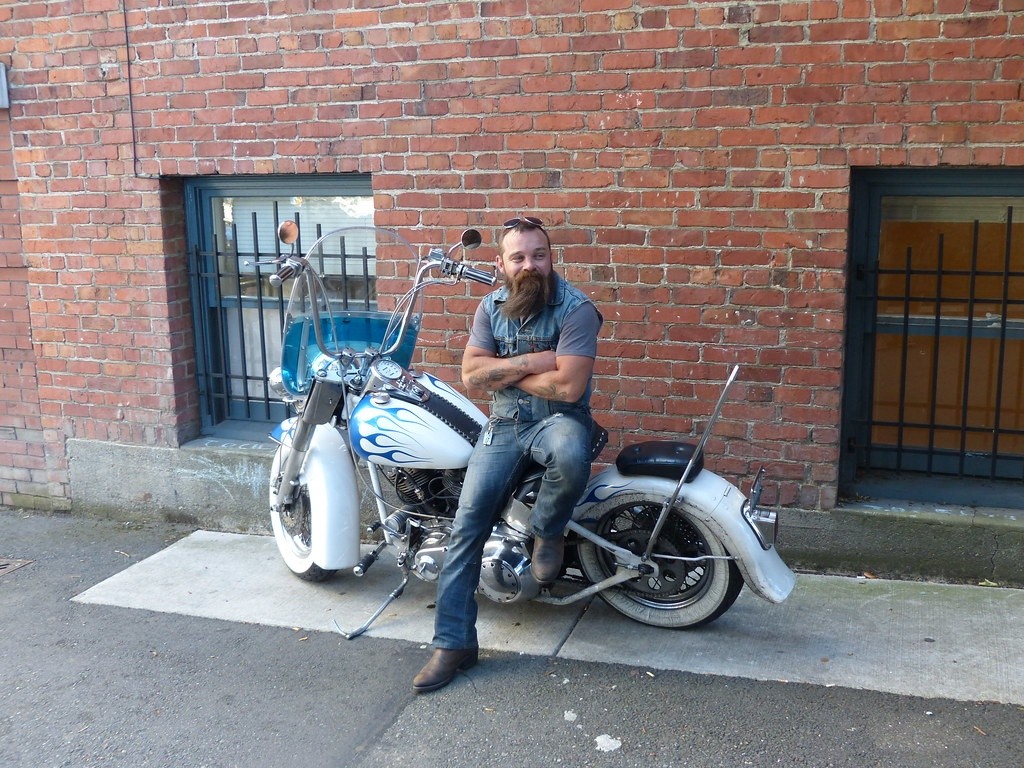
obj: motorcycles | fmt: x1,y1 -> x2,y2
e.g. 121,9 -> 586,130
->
242,219 -> 797,632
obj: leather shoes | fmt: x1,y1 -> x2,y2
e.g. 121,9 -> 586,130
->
413,648 -> 478,692
530,535 -> 565,583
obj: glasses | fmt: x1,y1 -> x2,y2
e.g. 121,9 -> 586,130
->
501,216 -> 544,232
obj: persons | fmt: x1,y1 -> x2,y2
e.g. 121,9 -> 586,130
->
413,216 -> 604,691
